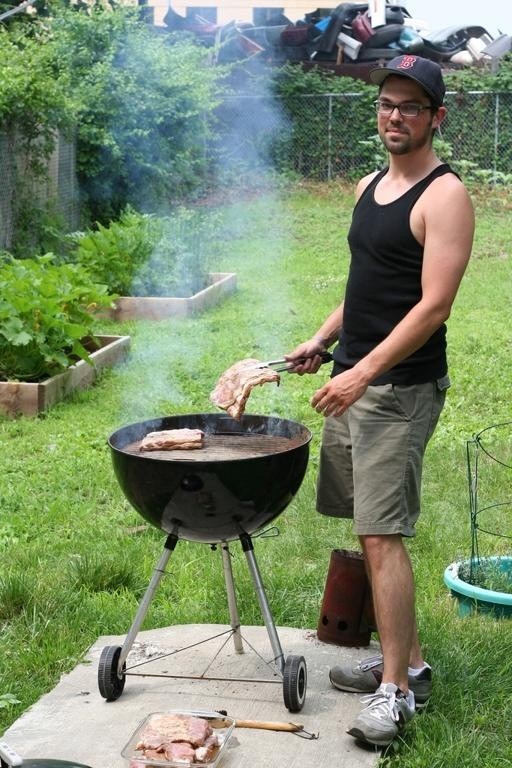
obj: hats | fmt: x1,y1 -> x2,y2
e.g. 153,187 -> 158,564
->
369,55 -> 446,105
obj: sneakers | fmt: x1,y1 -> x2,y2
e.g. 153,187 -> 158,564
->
329,652 -> 432,746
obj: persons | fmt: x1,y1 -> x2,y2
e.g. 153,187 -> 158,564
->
282,53 -> 475,749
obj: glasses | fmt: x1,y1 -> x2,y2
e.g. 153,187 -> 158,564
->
374,101 -> 433,117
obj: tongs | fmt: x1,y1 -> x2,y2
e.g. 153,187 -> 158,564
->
252,351 -> 334,373
